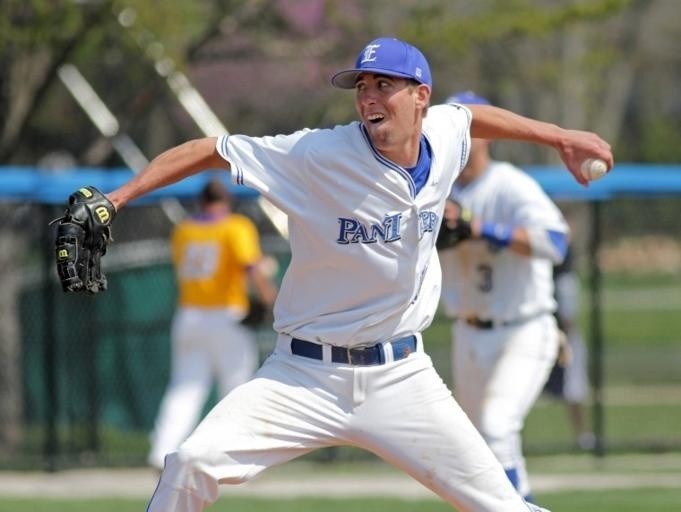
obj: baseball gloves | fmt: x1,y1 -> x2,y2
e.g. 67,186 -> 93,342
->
436,198 -> 471,249
54,186 -> 117,293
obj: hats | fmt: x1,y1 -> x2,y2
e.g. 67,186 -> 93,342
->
330,36 -> 434,91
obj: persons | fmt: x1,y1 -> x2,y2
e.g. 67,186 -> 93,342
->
50,37 -> 613,512
433,90 -> 572,509
145,176 -> 281,473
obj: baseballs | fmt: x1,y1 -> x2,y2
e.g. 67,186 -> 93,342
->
580,158 -> 607,180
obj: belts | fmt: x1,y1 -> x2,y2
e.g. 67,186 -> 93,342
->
276,333 -> 424,368
